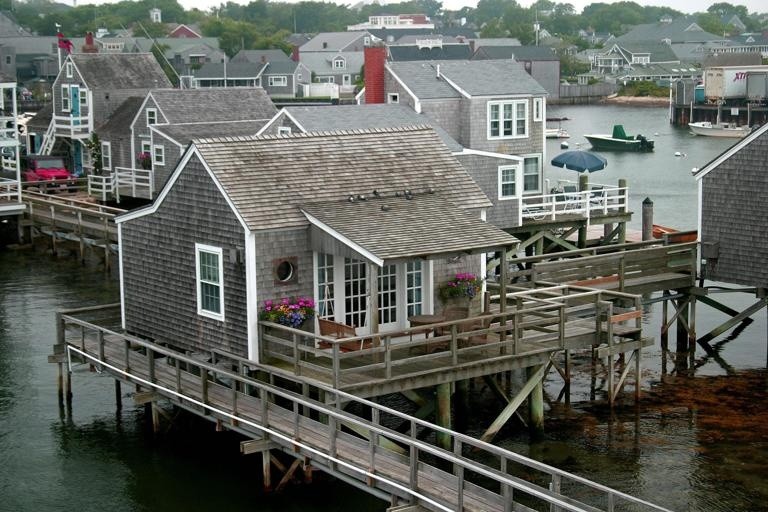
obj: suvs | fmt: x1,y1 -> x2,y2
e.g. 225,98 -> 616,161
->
19,156 -> 78,194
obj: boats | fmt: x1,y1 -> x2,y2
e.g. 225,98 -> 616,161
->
652,224 -> 698,243
544,127 -> 569,139
688,121 -> 752,139
584,125 -> 654,151
544,116 -> 570,121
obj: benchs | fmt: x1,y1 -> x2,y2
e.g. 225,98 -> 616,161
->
316,318 -> 379,350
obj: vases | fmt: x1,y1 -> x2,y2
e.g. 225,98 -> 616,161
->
278,315 -> 305,330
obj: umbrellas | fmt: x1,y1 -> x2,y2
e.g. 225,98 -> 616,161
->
551,149 -> 607,191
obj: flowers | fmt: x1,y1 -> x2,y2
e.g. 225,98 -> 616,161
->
260,295 -> 315,328
438,273 -> 486,304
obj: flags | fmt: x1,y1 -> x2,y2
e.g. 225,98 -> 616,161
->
58,31 -> 73,53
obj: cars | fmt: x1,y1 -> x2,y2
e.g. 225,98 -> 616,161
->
559,78 -> 568,86
7,85 -> 31,99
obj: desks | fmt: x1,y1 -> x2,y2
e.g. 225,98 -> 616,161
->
407,314 -> 445,357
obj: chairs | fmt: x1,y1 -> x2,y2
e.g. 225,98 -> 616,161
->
446,313 -> 495,358
561,183 -> 606,215
437,306 -> 470,348
